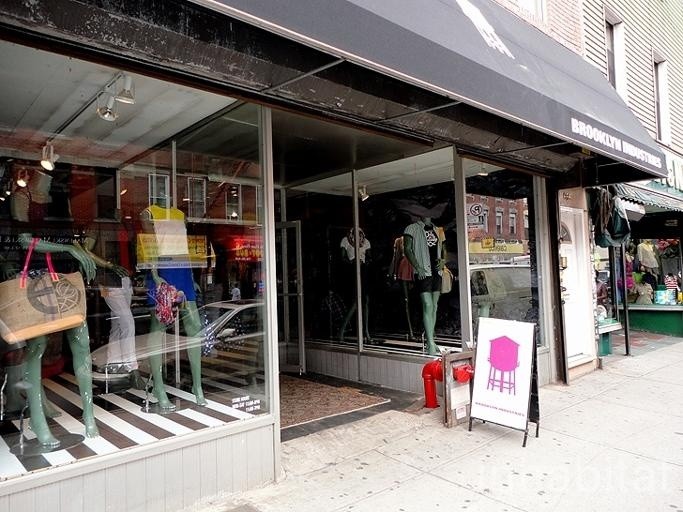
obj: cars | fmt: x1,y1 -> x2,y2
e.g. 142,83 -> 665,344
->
469,265 -> 531,312
78,299 -> 263,394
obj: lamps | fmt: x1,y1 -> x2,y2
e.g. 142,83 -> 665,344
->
1,165 -> 29,201
96,87 -> 119,121
41,141 -> 60,170
115,72 -> 136,103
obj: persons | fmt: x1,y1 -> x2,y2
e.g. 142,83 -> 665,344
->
84,207 -> 146,389
388,234 -> 418,340
337,224 -> 377,344
8,159 -> 99,449
230,283 -> 241,301
403,216 -> 447,356
626,239 -> 681,305
140,189 -> 208,409
595,270 -> 608,309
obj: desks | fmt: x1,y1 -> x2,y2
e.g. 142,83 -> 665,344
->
614,305 -> 683,337
596,319 -> 622,356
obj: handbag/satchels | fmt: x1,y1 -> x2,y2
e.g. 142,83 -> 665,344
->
0,271 -> 87,344
441,265 -> 453,293
200,313 -> 216,358
